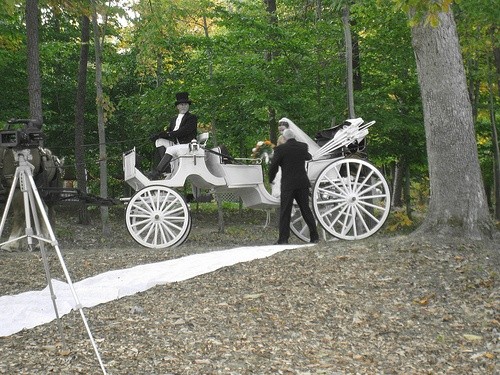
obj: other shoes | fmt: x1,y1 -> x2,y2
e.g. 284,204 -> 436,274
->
273,240 -> 288,245
309,240 -> 319,243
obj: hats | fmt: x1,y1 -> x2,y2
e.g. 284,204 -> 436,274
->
175,92 -> 192,105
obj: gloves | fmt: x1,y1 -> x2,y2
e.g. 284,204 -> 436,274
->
153,130 -> 168,141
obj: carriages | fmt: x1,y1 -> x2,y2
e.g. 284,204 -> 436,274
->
0,116 -> 391,251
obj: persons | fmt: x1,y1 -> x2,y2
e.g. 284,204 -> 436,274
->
144,92 -> 198,180
265,126 -> 320,246
259,117 -> 331,199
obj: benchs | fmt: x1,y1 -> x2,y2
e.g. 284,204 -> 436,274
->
302,137 -> 343,172
169,132 -> 210,162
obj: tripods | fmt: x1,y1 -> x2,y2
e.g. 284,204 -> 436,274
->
0,161 -> 106,375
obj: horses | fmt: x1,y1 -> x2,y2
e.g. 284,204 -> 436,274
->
0,146 -> 63,252
